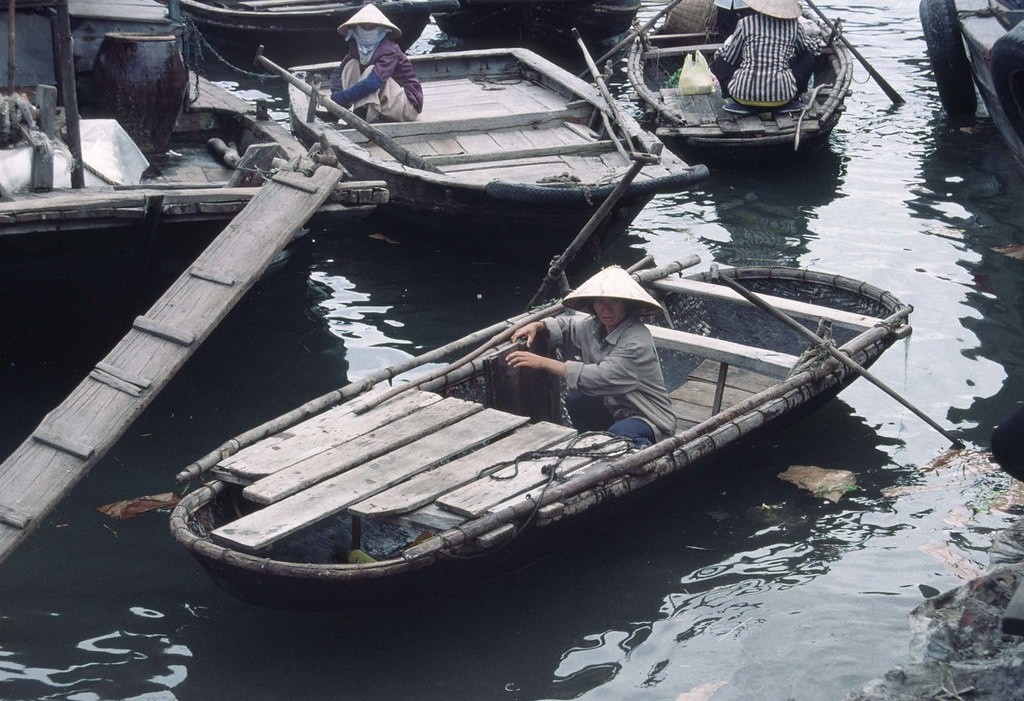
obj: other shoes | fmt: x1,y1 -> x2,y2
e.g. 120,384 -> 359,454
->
314,109 -> 338,123
778,101 -> 807,112
723,102 -> 750,114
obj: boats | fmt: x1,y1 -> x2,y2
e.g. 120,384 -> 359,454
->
468,0 -> 644,45
919,0 -> 1024,180
630,1 -> 855,156
180,0 -> 463,56
169,265 -> 914,600
287,47 -> 711,256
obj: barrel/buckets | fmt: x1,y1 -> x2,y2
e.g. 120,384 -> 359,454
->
89,30 -> 191,179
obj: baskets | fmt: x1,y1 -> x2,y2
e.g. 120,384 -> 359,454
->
663,0 -> 718,43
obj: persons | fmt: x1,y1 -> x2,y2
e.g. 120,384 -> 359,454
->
316,3 -> 423,131
506,264 -> 678,453
710,1 -> 822,114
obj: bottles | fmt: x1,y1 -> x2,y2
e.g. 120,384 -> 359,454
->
345,549 -> 377,564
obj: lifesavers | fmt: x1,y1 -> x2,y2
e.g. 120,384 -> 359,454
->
918,2 -> 982,121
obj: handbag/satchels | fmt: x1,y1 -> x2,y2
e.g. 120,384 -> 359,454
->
679,50 -> 713,95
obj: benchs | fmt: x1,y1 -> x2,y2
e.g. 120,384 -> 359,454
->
654,274 -> 885,332
561,312 -> 800,417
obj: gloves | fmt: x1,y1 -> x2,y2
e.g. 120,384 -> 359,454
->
331,70 -> 386,105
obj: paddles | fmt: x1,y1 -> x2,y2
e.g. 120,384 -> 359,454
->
571,26 -> 640,156
252,41 -> 446,173
807,0 -> 905,106
574,0 -> 684,79
524,149 -> 660,314
708,262 -> 965,453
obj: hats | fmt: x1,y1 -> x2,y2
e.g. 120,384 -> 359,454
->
562,264 -> 664,316
338,4 -> 402,40
743,0 -> 801,19
